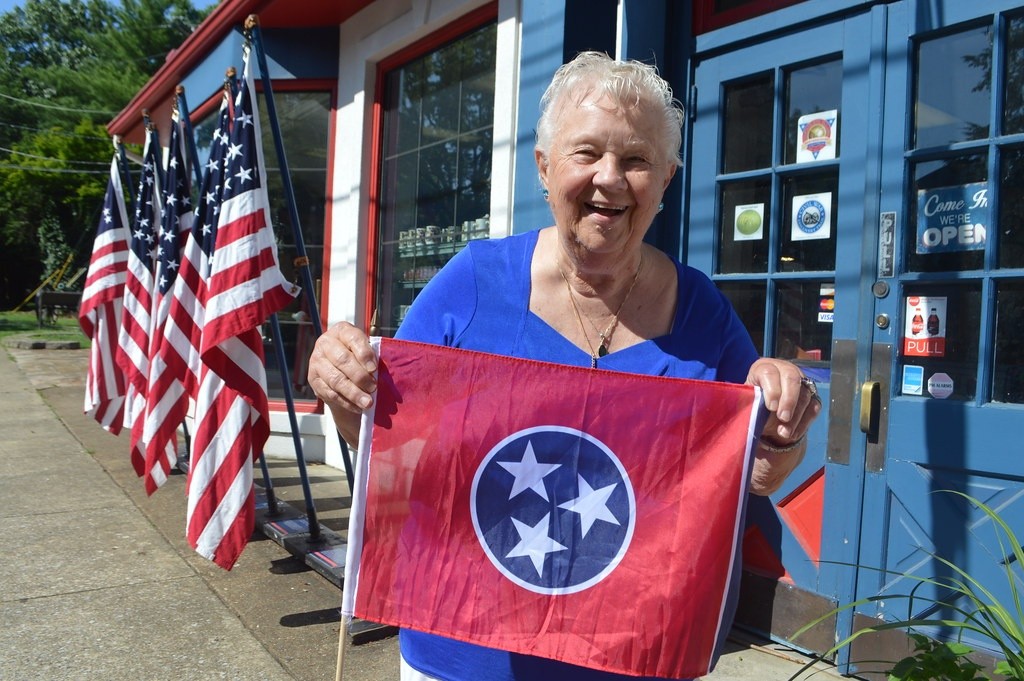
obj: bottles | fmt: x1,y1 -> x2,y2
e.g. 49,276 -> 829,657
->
927,308 -> 939,337
912,308 -> 924,335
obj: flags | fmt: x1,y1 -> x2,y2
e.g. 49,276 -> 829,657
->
347,336 -> 770,679
78,59 -> 303,572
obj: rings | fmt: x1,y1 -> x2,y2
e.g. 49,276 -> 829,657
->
813,395 -> 823,406
801,377 -> 818,398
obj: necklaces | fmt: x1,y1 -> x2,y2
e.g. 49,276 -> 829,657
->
551,247 -> 645,369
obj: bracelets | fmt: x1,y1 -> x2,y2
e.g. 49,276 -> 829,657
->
760,433 -> 805,454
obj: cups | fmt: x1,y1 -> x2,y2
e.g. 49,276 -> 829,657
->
398,219 -> 489,249
392,305 -> 410,319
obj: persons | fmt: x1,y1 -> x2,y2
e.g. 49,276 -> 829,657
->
308,51 -> 822,681
